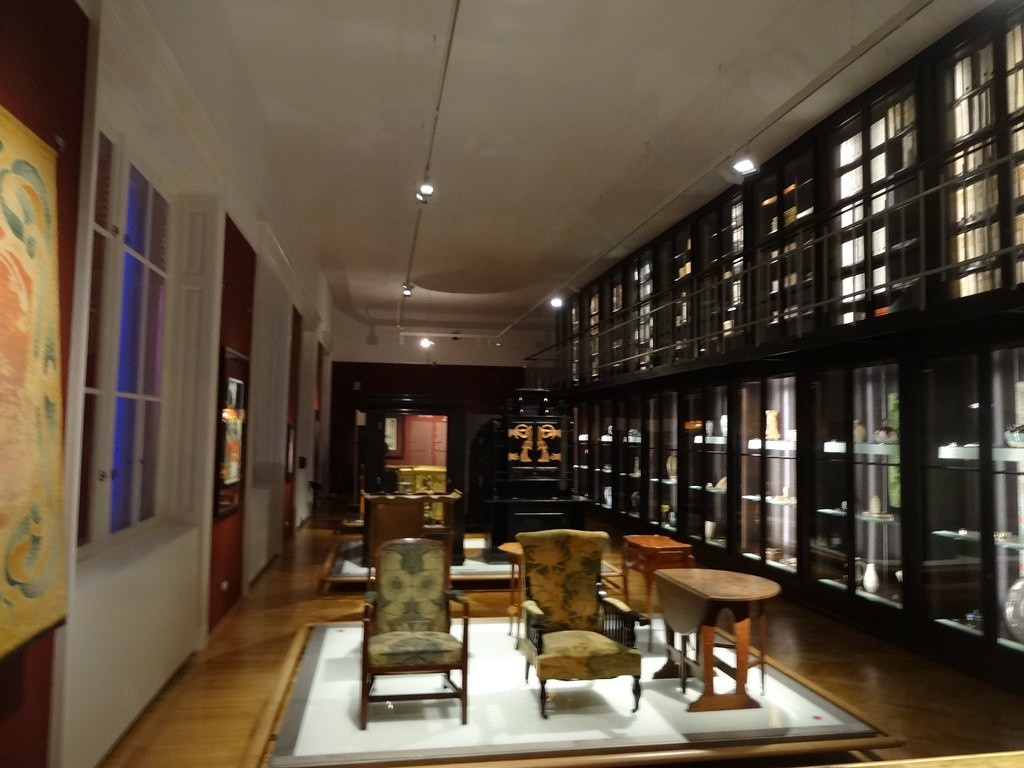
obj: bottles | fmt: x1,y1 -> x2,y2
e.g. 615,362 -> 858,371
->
870,495 -> 881,515
863,563 -> 879,592
853,420 -> 867,443
1003,382 -> 1024,449
872,420 -> 898,444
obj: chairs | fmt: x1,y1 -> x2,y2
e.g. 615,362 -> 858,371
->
361,538 -> 471,727
515,529 -> 644,717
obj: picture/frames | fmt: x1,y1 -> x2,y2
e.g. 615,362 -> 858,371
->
386,414 -> 405,458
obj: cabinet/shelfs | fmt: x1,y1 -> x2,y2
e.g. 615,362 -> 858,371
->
504,386 -> 569,481
561,19 -> 1024,648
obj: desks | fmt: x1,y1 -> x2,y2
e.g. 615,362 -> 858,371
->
652,568 -> 782,709
499,543 -> 533,644
622,532 -> 698,623
362,488 -> 464,567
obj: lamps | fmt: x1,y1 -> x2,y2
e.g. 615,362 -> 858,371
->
732,150 -> 760,179
401,177 -> 434,297
